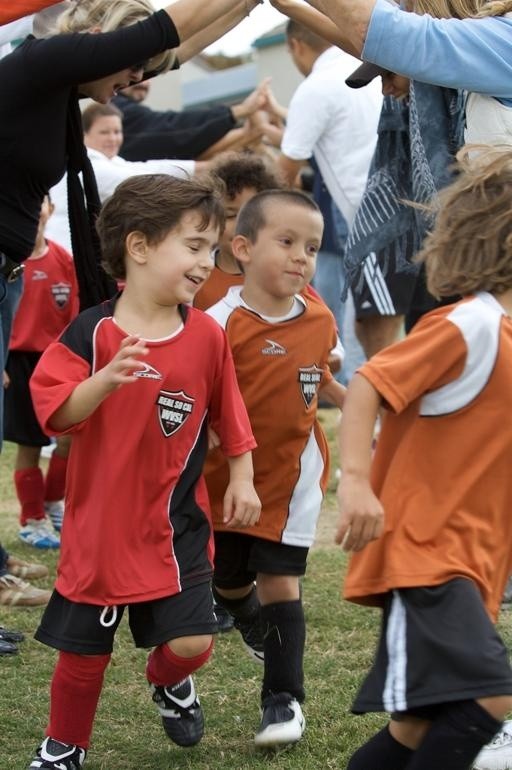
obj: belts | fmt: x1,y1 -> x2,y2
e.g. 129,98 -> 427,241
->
1,257 -> 26,284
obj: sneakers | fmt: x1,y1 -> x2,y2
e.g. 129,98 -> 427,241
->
213,602 -> 236,632
25,735 -> 89,770
255,697 -> 307,748
147,677 -> 208,745
2,554 -> 48,579
1,572 -> 53,606
235,607 -> 266,664
1,626 -> 24,656
18,500 -> 64,548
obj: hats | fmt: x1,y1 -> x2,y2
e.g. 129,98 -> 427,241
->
344,60 -> 385,89
